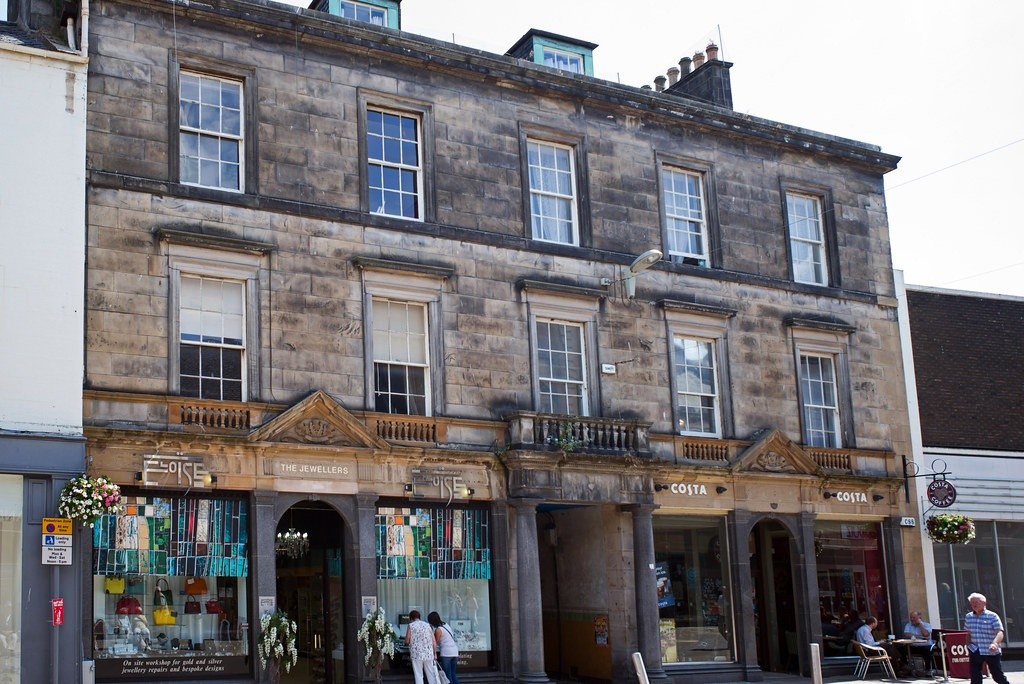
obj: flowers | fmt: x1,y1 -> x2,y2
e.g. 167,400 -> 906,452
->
58,475 -> 126,529
357,606 -> 399,666
257,608 -> 298,674
926,513 -> 976,545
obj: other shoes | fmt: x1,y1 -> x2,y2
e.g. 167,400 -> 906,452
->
896,670 -> 904,678
925,668 -> 931,676
900,664 -> 912,675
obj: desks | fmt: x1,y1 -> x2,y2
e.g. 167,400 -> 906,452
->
882,640 -> 933,678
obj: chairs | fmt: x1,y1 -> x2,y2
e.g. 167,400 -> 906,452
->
851,639 -> 897,680
909,639 -> 938,675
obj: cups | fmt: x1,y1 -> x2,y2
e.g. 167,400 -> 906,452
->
888,635 -> 895,640
657,577 -> 669,599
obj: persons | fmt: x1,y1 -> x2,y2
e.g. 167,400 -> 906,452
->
963,592 -> 1011,684
404,610 -> 441,684
820,607 -> 865,656
897,611 -> 934,678
857,617 -> 916,678
426,612 -> 461,684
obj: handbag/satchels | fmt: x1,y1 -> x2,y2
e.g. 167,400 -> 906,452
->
435,660 -> 451,684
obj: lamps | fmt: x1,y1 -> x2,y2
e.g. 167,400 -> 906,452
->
468,488 -> 475,495
405,484 -> 412,492
275,528 -> 309,559
601,249 -> 663,286
824,491 -> 837,500
211,474 -> 218,483
135,471 -> 142,481
655,484 -> 669,492
